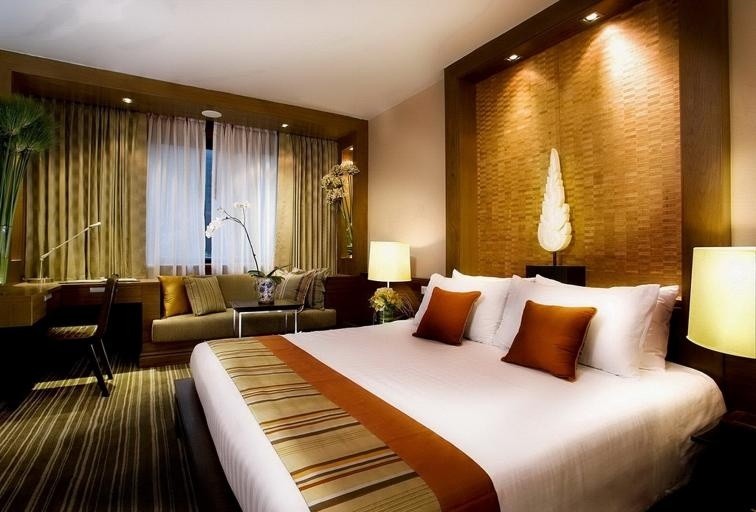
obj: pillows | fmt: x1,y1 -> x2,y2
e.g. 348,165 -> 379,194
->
411,269 -> 680,381
304,267 -> 330,310
181,275 -> 227,317
272,266 -> 315,312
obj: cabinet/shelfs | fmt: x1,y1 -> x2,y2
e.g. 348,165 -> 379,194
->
61,281 -> 161,368
0,284 -> 63,328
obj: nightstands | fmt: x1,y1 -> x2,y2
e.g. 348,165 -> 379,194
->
690,424 -> 755,511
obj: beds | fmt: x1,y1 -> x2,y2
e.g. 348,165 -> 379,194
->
174,300 -> 726,511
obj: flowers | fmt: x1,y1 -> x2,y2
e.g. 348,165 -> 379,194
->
368,287 -> 406,312
205,203 -> 293,284
320,159 -> 360,222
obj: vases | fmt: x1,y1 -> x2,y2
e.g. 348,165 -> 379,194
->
379,305 -> 395,323
342,222 -> 353,259
255,277 -> 276,305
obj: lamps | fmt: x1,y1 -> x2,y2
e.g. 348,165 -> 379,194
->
26,222 -> 102,283
367,240 -> 412,288
686,246 -> 756,432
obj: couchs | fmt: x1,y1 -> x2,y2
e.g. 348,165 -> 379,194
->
152,273 -> 354,368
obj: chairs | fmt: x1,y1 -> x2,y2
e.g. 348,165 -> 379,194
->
29,278 -> 118,397
